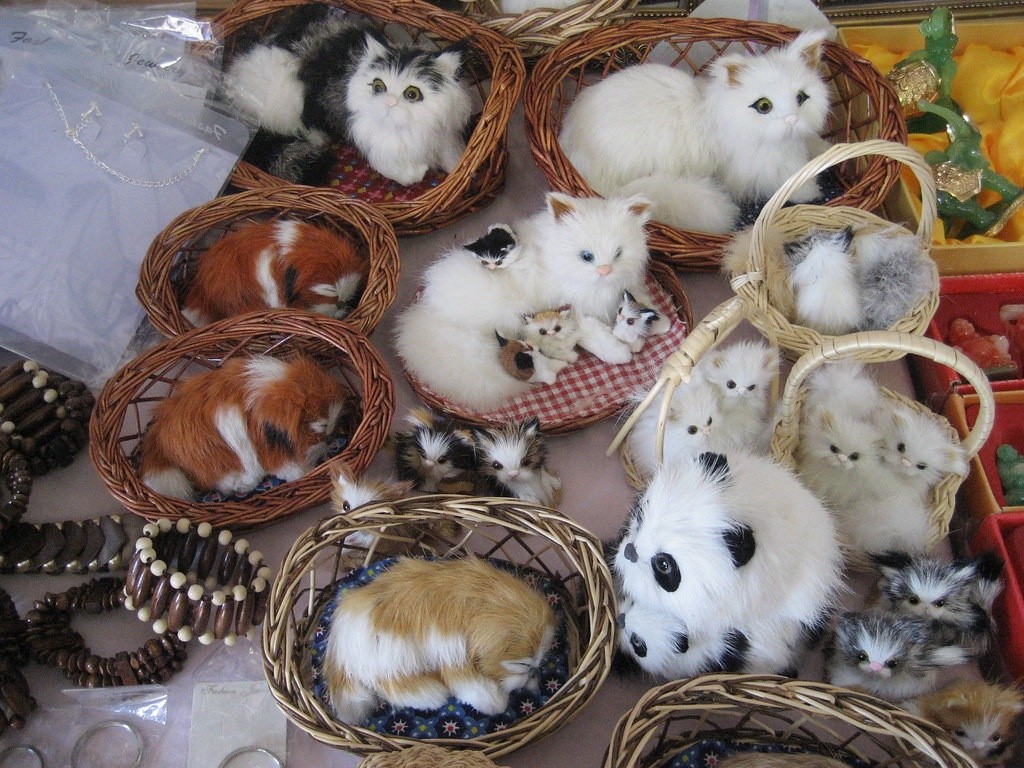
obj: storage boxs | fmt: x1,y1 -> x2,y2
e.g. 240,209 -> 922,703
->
941,392 -> 1024,540
968,511 -> 1024,681
828,20 -> 1024,276
910,271 -> 1024,411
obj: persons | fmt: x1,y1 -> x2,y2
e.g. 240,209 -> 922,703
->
996,444 -> 1024,505
948,317 -> 1018,371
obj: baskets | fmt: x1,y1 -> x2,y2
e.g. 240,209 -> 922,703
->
89,309 -> 393,535
191,0 -> 527,236
601,675 -> 978,768
392,247 -> 695,437
260,493 -> 620,759
765,331 -> 995,572
729,138 -> 942,367
422,0 -> 706,71
526,17 -> 907,273
137,184 -> 407,370
604,294 -> 780,498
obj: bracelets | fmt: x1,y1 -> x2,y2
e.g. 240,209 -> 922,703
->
0,358 -> 94,524
0,745 -> 45,768
122,518 -> 272,646
215,745 -> 285,768
69,718 -> 147,768
0,586 -> 40,735
18,575 -> 188,689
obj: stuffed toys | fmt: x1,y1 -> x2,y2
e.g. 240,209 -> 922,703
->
560,28 -> 844,236
225,6 -> 490,183
319,190 -> 1024,768
139,353 -> 351,505
196,220 -> 362,321
